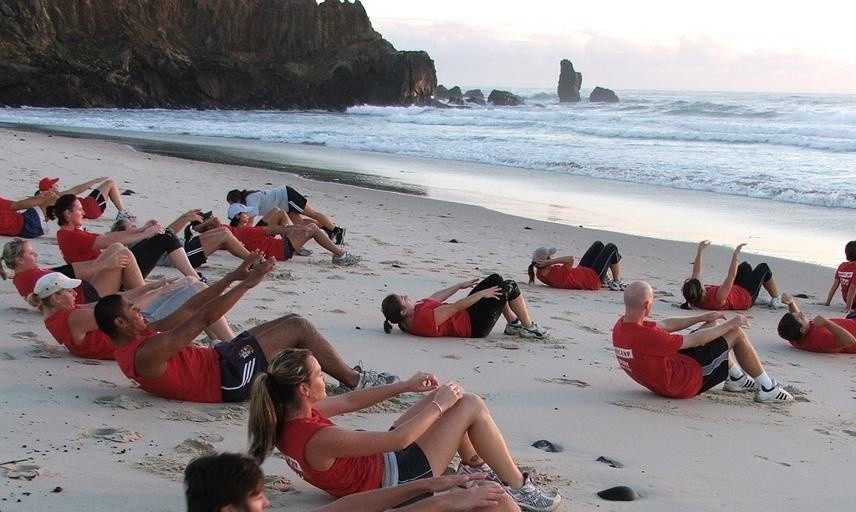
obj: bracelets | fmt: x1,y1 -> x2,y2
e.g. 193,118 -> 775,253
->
432,400 -> 443,417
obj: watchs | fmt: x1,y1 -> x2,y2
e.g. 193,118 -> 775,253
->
285,224 -> 288,229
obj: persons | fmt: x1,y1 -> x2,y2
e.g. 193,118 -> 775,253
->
184,210 -> 362,266
817,241 -> 856,314
249,349 -> 562,512
1,193 -> 90,239
381,273 -> 551,340
1,239 -> 146,310
680,241 -> 791,309
228,203 -> 313,256
183,451 -> 522,512
45,194 -> 209,290
227,183 -> 345,245
611,281 -> 793,403
778,292 -> 856,353
95,249 -> 399,403
35,176 -> 136,219
111,208 -> 280,270
27,271 -> 236,360
528,241 -> 631,291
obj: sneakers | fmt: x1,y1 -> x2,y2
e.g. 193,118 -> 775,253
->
455,461 -> 504,490
332,250 -> 361,266
295,248 -> 313,256
610,280 -> 629,291
116,209 -> 136,221
327,224 -> 341,240
352,364 -> 400,390
753,383 -> 793,403
334,227 -> 347,246
600,278 -> 612,288
723,374 -> 756,392
519,323 -> 550,339
770,295 -> 786,308
503,320 -> 521,336
500,472 -> 561,512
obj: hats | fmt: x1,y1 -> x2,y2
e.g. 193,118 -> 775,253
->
33,271 -> 82,299
191,211 -> 212,226
227,203 -> 253,220
39,177 -> 59,191
532,247 -> 556,264
